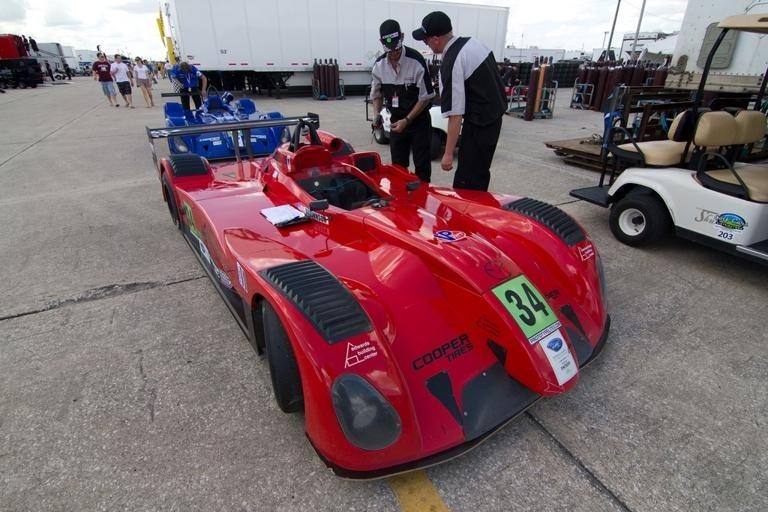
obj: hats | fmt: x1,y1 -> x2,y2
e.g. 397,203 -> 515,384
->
411,10 -> 452,41
378,18 -> 403,54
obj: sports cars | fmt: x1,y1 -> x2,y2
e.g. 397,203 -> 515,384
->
159,90 -> 291,162
141,113 -> 616,481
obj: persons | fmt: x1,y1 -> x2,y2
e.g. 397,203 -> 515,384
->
126,59 -> 166,87
64,63 -> 72,80
368,18 -> 437,184
28,36 -> 40,56
411,11 -> 508,192
171,61 -> 207,122
21,35 -> 31,57
133,57 -> 155,108
163,59 -> 174,84
110,54 -> 135,109
91,52 -> 120,109
44,59 -> 55,81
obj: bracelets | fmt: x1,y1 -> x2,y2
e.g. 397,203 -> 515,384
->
405,115 -> 410,125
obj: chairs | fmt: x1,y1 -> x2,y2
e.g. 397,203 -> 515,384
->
697,110 -> 768,204
699,106 -> 745,158
733,112 -> 768,170
616,107 -> 711,169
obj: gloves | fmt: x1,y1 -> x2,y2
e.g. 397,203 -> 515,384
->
370,114 -> 390,146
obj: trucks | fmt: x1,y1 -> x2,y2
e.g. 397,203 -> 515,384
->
0,33 -> 133,90
